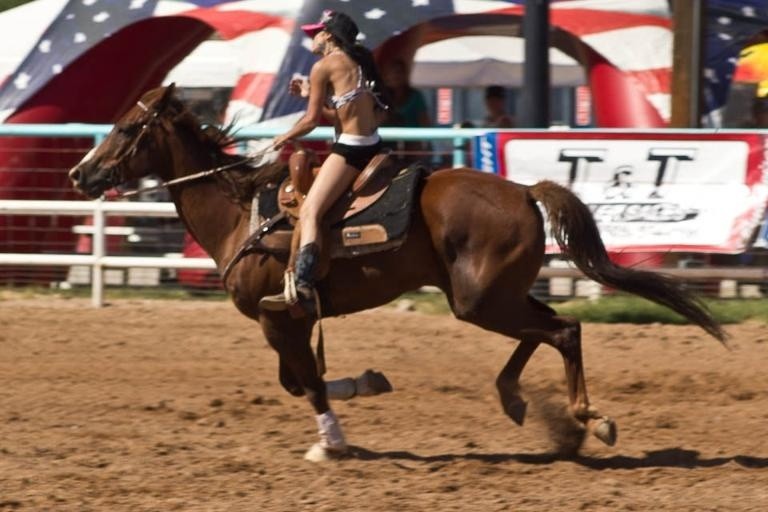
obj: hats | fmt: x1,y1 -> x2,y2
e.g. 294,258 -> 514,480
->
300,9 -> 358,43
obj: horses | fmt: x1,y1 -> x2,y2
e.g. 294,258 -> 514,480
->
66,80 -> 735,463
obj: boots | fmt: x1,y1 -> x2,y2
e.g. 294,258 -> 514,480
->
259,243 -> 320,314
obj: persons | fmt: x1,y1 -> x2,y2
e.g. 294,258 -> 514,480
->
382,58 -> 430,125
257,7 -> 386,315
475,83 -> 515,126
